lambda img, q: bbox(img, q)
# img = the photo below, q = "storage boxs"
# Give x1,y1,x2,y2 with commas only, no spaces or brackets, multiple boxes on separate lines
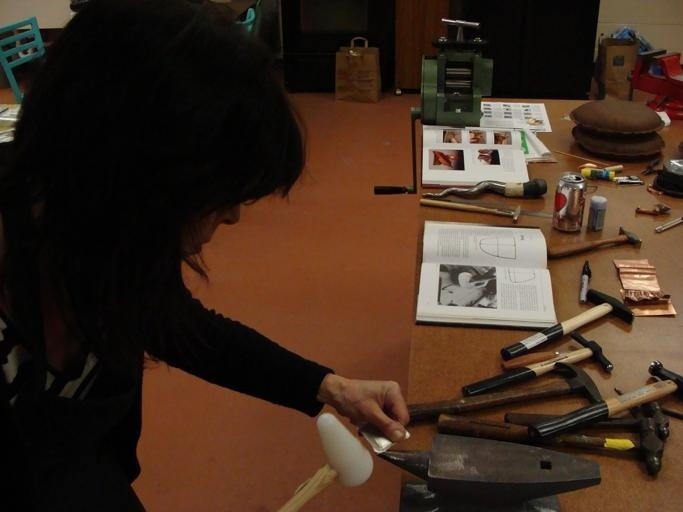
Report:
592,33,683,119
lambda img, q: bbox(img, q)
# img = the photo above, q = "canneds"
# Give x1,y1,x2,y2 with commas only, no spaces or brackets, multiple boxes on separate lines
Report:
552,172,587,232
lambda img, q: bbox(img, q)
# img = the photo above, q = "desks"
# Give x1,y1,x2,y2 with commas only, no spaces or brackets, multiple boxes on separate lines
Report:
404,98,682,510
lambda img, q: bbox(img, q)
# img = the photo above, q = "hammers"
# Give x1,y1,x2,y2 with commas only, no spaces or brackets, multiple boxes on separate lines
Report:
545,226,643,259
500,287,635,362
388,332,683,478
419,197,522,223
277,411,374,512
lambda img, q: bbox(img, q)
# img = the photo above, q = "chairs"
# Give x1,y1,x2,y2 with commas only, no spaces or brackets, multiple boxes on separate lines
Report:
0,16,52,101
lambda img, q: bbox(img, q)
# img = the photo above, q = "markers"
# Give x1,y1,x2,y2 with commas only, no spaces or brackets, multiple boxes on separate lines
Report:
580,259,591,302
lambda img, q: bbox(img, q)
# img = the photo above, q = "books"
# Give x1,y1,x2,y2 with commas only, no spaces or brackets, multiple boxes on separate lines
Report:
416,220,559,330
421,123,530,189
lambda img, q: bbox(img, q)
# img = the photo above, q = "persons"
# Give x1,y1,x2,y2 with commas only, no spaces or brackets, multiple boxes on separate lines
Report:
0,1,415,512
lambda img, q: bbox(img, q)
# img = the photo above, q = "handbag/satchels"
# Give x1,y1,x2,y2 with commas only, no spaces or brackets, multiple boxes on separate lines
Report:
598,32,640,102
334,36,382,104
